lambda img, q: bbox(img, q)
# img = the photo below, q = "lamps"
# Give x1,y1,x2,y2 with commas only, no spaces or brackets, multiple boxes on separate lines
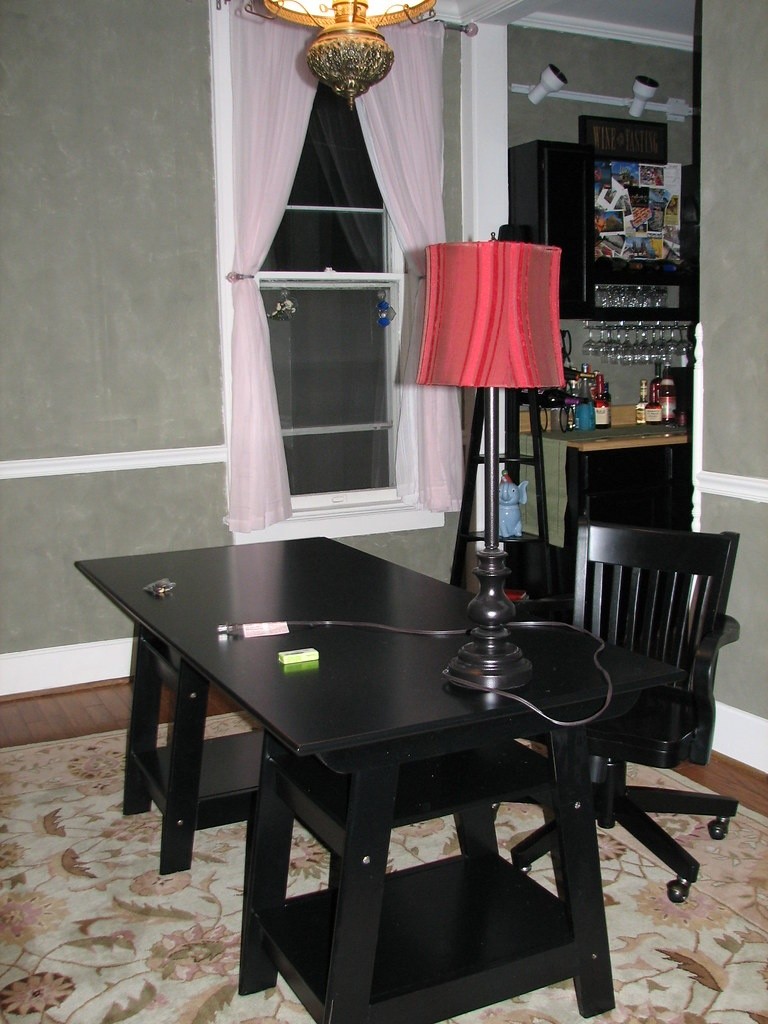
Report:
414,234,565,695
529,64,568,105
629,76,659,116
215,0,439,110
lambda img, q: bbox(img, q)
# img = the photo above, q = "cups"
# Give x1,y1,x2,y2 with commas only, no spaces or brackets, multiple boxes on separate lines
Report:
595,285,668,307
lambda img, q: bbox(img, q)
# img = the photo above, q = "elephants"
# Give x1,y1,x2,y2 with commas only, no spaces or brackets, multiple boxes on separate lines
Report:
498,480,529,538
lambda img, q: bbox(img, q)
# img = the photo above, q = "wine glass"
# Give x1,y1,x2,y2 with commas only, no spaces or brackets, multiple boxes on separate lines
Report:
581,320,693,366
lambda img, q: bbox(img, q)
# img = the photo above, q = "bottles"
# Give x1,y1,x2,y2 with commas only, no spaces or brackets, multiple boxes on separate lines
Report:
650,363,662,404
594,374,609,429
660,364,677,425
636,379,648,424
574,364,596,431
645,382,663,425
603,380,611,427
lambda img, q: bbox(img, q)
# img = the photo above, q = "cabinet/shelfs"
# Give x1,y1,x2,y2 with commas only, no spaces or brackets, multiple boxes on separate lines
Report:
509,137,700,319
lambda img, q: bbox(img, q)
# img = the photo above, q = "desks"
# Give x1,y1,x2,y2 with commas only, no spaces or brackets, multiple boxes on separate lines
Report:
73,534,616,1024
512,428,688,555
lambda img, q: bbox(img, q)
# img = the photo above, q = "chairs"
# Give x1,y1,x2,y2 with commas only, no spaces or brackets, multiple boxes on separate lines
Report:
508,520,740,903
